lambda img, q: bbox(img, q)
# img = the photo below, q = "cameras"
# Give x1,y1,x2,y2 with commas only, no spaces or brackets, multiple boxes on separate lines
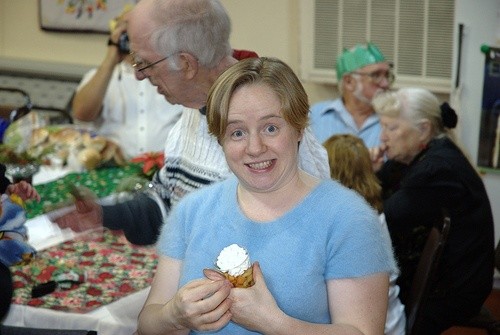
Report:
118,31,130,55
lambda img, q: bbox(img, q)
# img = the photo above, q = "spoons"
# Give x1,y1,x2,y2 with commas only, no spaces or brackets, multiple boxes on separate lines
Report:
203,268,234,288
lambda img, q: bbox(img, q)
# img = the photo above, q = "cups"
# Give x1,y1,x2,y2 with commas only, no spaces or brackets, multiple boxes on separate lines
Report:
214,253,255,288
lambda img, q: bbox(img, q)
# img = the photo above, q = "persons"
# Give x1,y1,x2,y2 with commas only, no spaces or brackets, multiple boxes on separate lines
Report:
320,132,407,334
136,56,398,334
364,85,494,334
72,7,188,163
305,40,396,164
42,1,331,248
0,160,42,205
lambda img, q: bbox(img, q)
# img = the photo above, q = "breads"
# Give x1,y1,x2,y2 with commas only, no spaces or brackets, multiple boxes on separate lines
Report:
12,109,127,170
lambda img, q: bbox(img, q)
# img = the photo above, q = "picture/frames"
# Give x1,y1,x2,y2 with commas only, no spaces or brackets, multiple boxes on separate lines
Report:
37,0,146,34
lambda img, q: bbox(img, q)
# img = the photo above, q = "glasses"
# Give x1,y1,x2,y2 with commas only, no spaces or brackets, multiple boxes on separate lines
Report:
346,69,394,82
128,50,180,73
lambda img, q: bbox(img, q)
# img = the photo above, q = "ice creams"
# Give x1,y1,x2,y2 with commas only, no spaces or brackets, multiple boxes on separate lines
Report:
216,243,253,288
